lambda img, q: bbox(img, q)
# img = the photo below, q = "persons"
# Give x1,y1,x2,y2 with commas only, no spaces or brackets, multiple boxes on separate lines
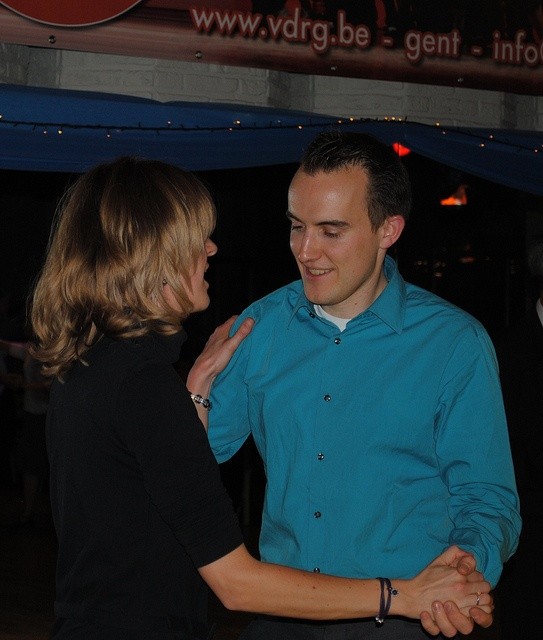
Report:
29,154,494,640
208,131,523,640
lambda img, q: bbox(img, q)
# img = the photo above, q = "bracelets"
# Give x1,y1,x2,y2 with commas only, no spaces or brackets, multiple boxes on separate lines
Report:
185,388,212,410
377,577,393,628
